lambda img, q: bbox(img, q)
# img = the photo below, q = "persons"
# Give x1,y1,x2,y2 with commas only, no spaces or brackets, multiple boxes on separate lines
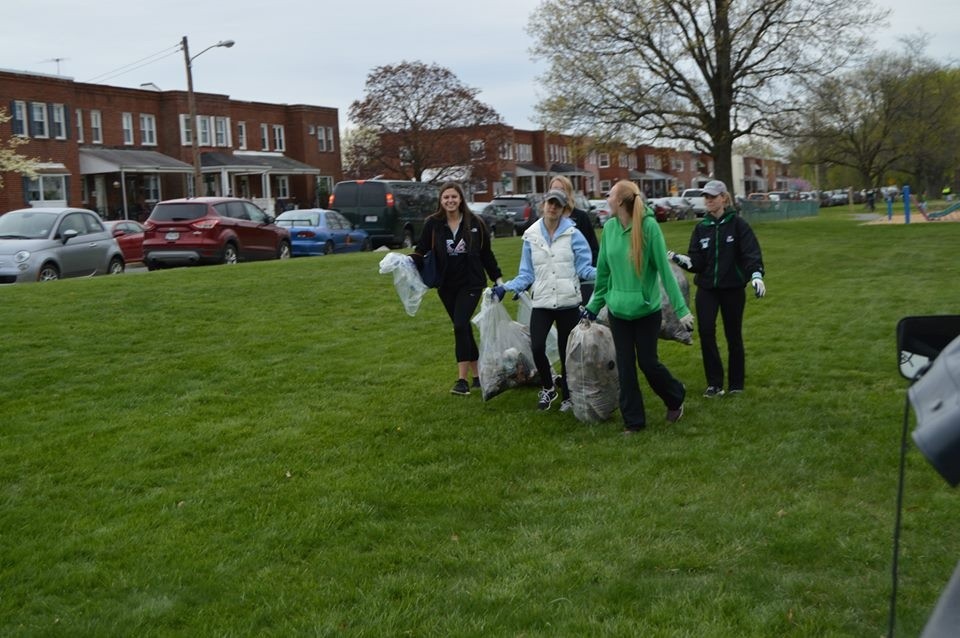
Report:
491,176,765,432
389,183,506,395
866,189,876,212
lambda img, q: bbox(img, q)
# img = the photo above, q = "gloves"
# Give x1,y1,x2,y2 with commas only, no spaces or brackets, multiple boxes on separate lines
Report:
491,286,506,302
752,278,766,298
667,251,683,265
581,309,596,320
678,313,695,331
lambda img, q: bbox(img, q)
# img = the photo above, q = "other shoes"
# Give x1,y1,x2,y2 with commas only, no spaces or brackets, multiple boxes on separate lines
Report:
666,383,685,423
622,426,633,433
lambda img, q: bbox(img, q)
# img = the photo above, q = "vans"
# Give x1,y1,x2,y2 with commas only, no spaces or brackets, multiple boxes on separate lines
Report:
327,179,442,250
681,189,707,215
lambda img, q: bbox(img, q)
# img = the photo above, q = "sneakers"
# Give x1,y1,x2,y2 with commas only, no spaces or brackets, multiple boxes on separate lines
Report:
730,389,742,394
703,386,725,398
472,382,480,388
537,385,559,411
450,379,471,395
560,398,573,412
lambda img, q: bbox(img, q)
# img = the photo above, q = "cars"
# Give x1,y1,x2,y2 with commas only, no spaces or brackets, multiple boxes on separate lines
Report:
491,195,541,236
466,202,517,238
103,219,145,263
274,207,371,256
747,193,772,212
768,191,849,211
647,198,697,222
587,200,613,228
143,196,292,272
0,208,125,284
576,195,598,226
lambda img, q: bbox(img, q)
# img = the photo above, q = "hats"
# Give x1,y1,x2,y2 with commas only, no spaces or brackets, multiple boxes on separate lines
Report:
543,189,569,207
700,180,727,196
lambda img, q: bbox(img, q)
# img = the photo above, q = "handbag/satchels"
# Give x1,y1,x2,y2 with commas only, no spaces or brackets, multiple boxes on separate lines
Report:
421,229,439,289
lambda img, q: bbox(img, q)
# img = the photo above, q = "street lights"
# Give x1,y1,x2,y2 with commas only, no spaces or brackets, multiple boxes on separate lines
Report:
185,40,235,197
